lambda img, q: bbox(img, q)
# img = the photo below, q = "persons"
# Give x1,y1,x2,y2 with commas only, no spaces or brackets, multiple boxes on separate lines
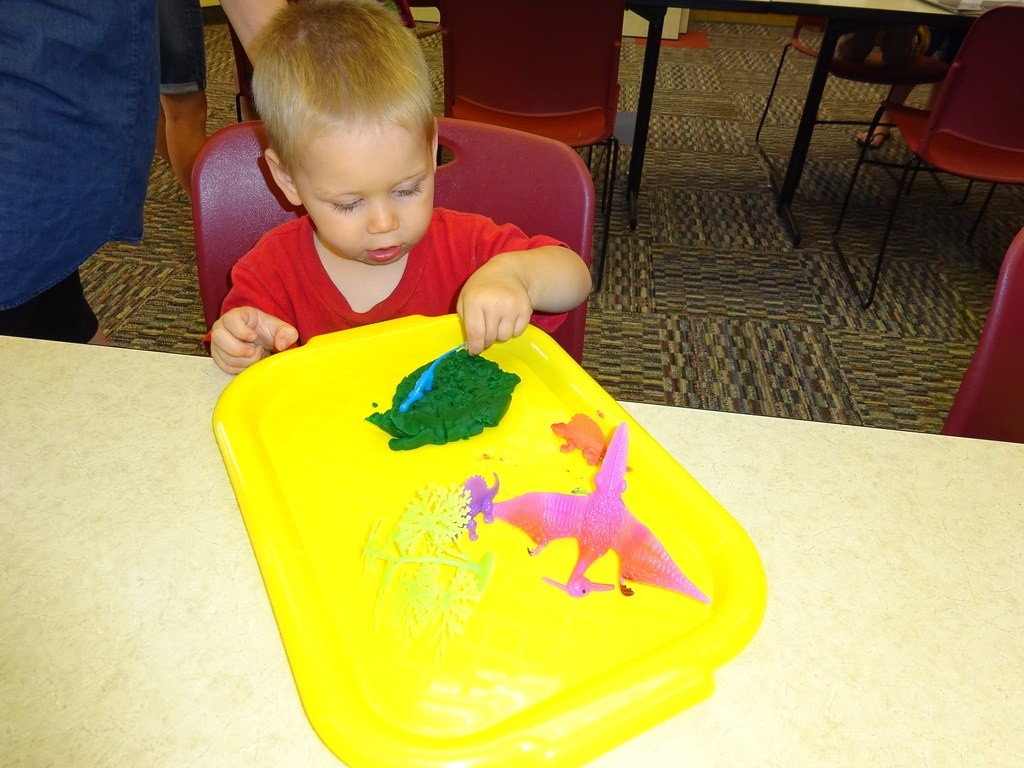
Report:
211,0,593,377
0,0,290,344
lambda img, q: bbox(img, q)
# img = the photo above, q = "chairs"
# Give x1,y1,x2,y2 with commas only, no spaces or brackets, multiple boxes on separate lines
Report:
190,0,1024,443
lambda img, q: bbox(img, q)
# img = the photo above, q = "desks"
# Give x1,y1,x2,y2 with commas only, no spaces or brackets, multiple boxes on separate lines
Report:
0,327,1024,768
618,0,981,243
